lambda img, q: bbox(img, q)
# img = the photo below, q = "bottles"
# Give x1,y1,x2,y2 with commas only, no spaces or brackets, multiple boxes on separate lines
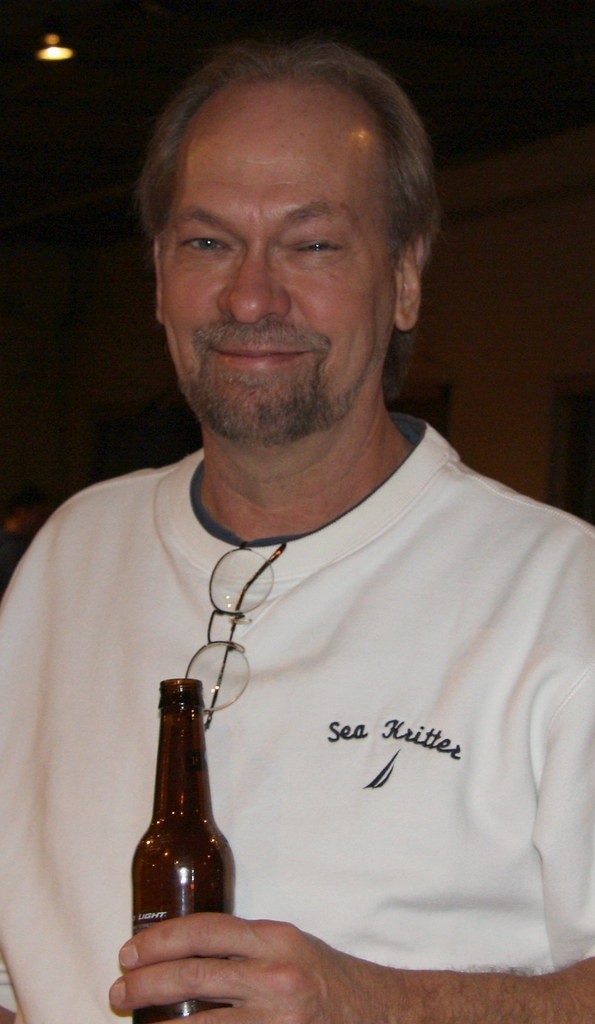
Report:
128,678,237,1024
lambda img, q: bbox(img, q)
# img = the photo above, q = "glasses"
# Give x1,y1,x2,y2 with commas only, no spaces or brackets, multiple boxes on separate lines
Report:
184,538,288,730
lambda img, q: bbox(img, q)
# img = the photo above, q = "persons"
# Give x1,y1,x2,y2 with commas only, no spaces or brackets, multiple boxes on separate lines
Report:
0,38,595,1024
0,490,46,598
